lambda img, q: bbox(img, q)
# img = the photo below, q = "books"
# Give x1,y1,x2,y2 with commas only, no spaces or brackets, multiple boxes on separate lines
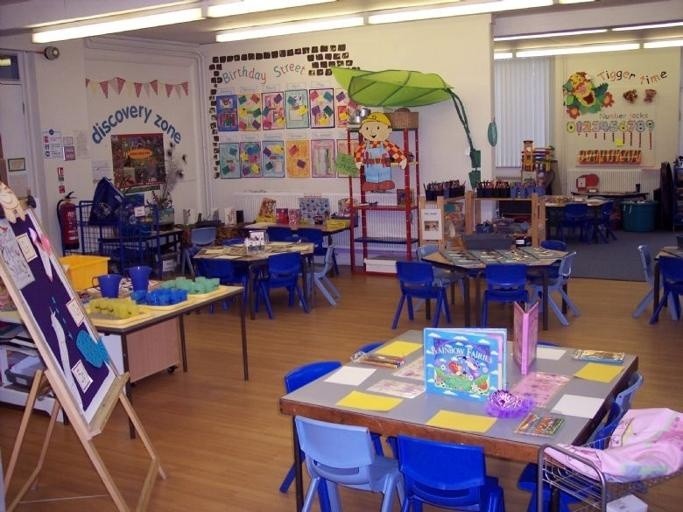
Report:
513,301,539,376
423,326,508,404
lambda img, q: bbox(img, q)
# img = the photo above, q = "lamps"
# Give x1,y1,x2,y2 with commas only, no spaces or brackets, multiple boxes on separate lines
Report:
27,0,366,43
370,0,591,27
493,24,682,61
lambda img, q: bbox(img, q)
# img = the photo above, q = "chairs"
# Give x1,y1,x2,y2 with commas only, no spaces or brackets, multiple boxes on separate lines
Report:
516,370,645,509
185,227,341,321
357,342,383,355
392,236,580,325
546,197,618,245
280,361,506,512
634,245,683,322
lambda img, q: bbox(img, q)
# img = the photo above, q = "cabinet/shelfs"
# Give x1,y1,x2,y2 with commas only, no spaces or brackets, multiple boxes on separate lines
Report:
342,125,419,270
98,226,182,280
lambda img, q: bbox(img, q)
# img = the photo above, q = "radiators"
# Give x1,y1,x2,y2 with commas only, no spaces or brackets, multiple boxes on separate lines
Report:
564,169,644,196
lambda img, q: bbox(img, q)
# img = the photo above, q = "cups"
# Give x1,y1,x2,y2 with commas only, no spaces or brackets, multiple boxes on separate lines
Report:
91,275,121,298
635,183,639,192
129,287,187,305
510,187,544,201
443,188,449,198
160,276,220,294
123,266,151,292
89,298,136,319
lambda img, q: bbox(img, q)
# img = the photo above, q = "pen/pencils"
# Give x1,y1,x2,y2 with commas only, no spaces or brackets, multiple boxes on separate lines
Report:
476,180,507,189
360,357,404,369
423,180,466,191
518,412,564,436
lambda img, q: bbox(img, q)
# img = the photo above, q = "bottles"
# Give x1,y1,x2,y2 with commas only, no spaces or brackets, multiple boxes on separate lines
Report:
516,236,525,250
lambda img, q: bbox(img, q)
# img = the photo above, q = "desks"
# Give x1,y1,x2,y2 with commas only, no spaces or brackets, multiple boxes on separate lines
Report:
0,277,248,439
279,330,639,512
244,217,351,275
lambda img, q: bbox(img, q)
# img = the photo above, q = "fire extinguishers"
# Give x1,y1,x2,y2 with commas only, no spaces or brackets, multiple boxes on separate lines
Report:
56,191,80,250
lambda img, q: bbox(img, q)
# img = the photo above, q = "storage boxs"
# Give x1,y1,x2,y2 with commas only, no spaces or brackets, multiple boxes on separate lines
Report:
57,253,109,292
